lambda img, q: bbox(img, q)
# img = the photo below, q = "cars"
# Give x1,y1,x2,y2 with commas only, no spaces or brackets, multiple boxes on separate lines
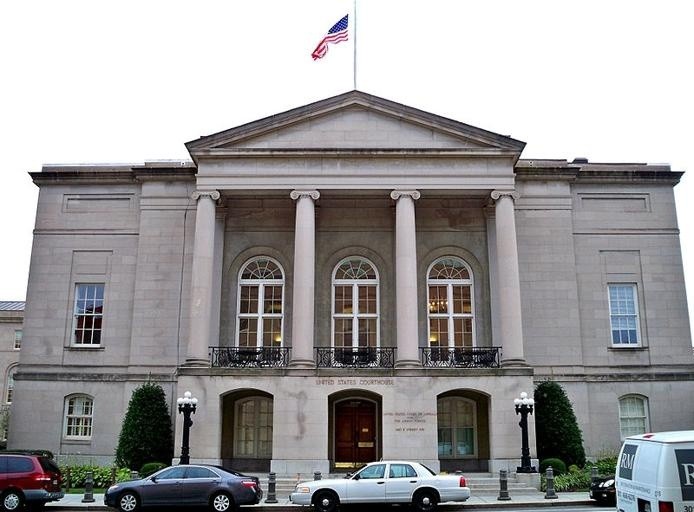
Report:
103,464,263,512
590,476,616,506
290,460,470,510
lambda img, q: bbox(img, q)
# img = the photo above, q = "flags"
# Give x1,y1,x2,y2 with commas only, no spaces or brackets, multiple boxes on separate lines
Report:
311,13,349,61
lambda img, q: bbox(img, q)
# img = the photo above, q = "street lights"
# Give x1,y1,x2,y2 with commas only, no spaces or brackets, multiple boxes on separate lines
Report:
176,392,198,464
513,392,534,466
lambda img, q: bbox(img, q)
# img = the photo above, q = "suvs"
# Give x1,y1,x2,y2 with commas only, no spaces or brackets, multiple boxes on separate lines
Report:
0,452,65,511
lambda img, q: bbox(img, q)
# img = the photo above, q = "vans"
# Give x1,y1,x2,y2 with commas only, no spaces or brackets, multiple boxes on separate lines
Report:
614,430,694,511
